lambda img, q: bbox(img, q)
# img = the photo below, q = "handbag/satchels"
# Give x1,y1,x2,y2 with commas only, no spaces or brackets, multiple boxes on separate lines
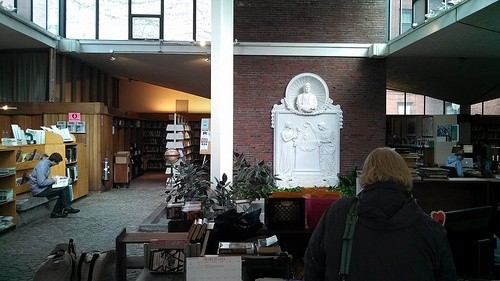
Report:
212,207,264,242
73,250,116,281
33,239,78,281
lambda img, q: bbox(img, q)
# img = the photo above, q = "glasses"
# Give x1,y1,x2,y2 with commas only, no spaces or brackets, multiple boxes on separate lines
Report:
54,161,59,164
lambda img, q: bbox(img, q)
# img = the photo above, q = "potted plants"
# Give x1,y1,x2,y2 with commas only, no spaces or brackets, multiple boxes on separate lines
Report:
166,151,283,224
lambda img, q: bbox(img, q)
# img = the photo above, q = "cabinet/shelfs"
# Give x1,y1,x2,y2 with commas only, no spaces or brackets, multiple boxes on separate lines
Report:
143,121,206,169
126,120,143,176
115,228,190,281
387,143,433,167
114,164,132,188
164,114,192,192
0,130,90,236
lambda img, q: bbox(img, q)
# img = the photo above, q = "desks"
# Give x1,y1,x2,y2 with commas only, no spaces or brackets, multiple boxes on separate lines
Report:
394,166,500,281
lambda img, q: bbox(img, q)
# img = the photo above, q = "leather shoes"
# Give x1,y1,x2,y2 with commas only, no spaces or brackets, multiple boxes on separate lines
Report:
64,207,80,214
51,212,67,217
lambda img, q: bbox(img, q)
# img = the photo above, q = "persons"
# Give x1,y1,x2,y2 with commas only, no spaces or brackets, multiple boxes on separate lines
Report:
475,155,492,170
307,121,335,179
296,82,317,113
279,121,298,180
30,153,80,219
304,147,448,281
445,153,464,176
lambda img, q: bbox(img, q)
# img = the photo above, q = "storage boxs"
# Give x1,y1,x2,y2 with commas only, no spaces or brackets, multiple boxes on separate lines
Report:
307,198,339,230
264,193,307,229
116,151,131,164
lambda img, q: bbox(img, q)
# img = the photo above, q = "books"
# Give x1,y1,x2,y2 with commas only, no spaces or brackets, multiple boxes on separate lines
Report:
0,187,14,202
165,200,202,220
142,121,166,167
178,121,209,161
187,218,208,240
0,167,16,177
393,148,450,180
69,185,73,201
65,147,78,181
40,124,74,142
1,125,46,146
219,235,280,256
52,176,69,187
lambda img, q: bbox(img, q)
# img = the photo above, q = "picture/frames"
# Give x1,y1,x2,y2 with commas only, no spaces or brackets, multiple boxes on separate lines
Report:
393,117,460,142
